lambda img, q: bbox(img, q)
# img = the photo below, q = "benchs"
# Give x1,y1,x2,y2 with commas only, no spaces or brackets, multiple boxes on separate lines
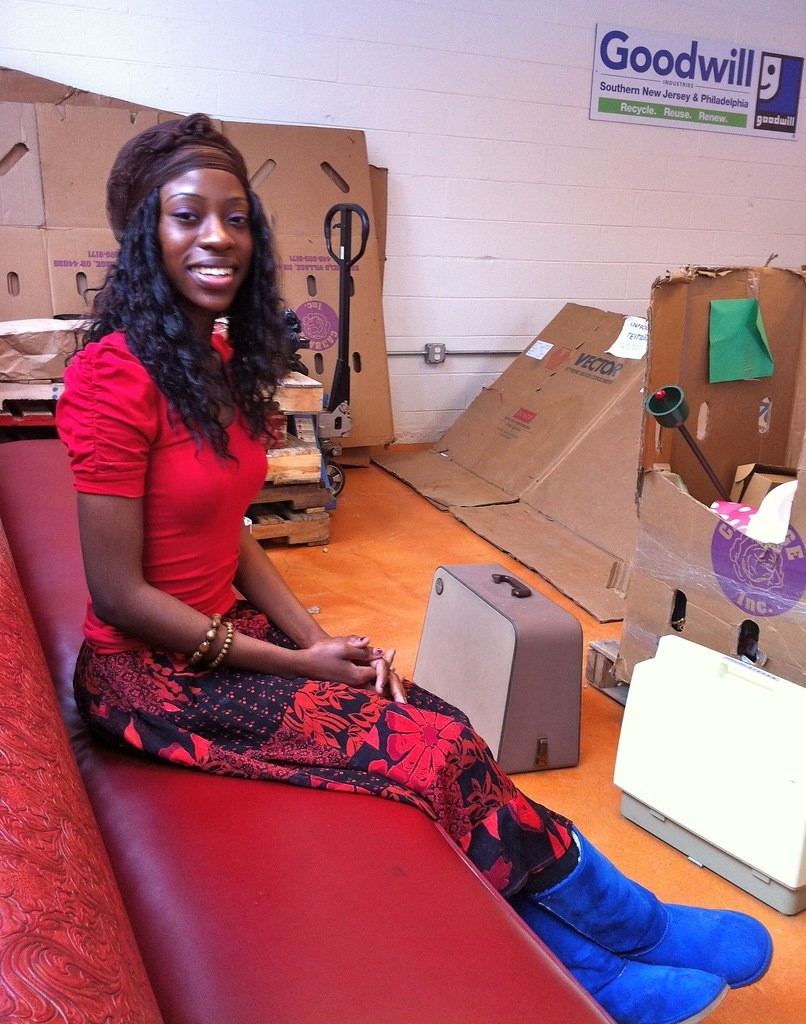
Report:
0,435,622,1024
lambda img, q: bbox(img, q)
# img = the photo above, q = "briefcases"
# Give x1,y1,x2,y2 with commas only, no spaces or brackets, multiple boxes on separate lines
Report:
413,564,584,773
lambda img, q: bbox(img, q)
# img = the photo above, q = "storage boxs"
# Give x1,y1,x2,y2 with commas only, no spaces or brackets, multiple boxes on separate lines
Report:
612,462,806,687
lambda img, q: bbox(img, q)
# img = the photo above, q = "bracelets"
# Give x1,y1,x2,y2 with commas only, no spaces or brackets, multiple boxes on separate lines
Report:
190,611,234,669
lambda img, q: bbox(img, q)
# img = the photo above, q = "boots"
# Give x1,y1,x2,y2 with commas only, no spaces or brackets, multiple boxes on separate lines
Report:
516,902,729,1024
532,825,774,988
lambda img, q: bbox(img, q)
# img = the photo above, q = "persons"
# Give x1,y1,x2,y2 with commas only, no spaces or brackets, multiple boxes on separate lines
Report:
55,113,775,1024
